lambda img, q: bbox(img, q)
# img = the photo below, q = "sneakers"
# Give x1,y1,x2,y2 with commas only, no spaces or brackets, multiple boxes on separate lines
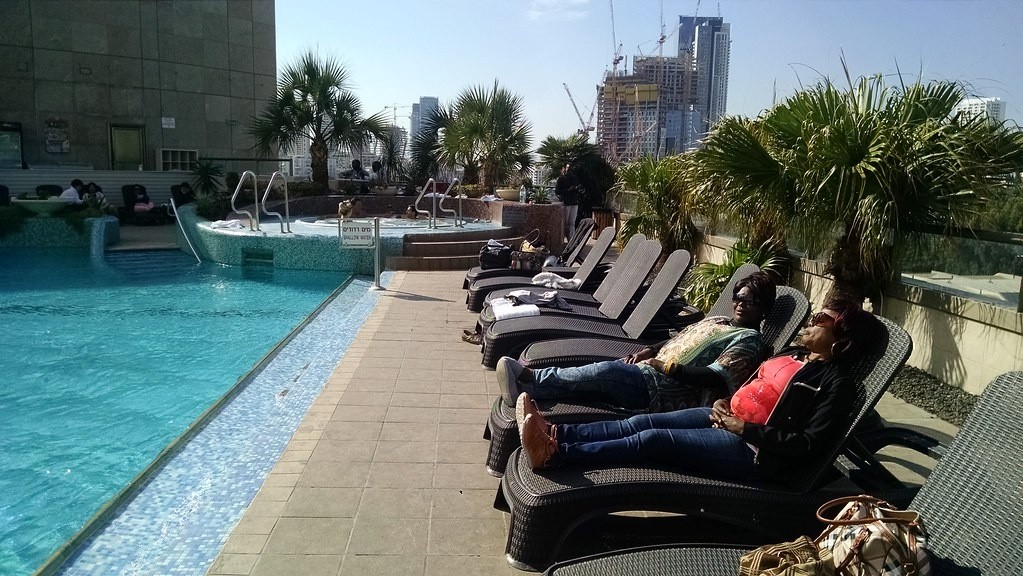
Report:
519,413,555,469
515,391,549,448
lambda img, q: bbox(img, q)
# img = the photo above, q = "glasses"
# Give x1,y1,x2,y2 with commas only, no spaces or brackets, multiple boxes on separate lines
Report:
811,312,835,326
730,294,761,306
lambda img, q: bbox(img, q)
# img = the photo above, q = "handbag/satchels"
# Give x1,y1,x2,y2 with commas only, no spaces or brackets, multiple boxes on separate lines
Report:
520,229,551,254
479,243,513,269
510,250,542,271
814,492,931,576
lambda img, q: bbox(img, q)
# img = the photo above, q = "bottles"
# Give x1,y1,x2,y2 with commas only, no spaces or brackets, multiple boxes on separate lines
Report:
519,184,526,203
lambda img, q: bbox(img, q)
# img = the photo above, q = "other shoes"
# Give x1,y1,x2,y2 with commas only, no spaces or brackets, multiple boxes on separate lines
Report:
461,324,483,344
500,356,527,385
496,358,517,407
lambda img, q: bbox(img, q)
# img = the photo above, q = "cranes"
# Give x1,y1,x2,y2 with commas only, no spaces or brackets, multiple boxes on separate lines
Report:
558,0,724,142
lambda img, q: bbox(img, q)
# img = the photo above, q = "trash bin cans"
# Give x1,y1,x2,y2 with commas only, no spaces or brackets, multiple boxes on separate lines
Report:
591,206,614,241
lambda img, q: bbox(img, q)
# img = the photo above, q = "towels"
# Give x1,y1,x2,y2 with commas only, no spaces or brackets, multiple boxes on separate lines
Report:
489,295,542,321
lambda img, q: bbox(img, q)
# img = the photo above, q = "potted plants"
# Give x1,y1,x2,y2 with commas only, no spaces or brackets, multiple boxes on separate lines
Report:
243,174,258,203
465,183,485,198
496,177,520,200
372,182,397,196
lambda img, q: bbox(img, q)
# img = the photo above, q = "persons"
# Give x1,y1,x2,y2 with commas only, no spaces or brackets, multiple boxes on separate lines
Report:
515,296,873,485
337,197,419,223
494,271,779,413
124,185,155,212
309,163,315,183
337,159,370,180
365,161,386,181
81,181,118,217
554,163,585,242
168,182,193,215
58,179,85,206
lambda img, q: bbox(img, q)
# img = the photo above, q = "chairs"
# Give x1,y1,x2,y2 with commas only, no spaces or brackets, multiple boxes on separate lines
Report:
463,217,599,290
465,225,617,313
492,312,915,574
36,184,63,196
475,240,664,342
516,263,761,370
482,233,647,307
483,285,812,478
171,184,194,197
480,248,691,369
121,184,166,226
542,366,1023,576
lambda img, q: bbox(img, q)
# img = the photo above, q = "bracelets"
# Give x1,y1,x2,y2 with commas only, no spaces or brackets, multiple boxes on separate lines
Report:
660,362,674,376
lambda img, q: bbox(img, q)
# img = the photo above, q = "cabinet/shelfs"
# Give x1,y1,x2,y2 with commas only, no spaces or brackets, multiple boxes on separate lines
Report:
154,147,199,172
